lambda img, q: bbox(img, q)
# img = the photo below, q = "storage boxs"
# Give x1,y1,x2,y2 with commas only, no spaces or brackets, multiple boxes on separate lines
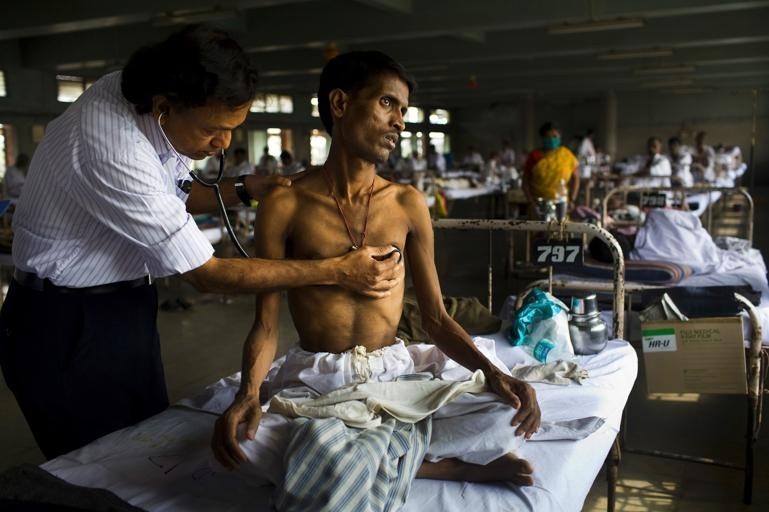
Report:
641,315,749,397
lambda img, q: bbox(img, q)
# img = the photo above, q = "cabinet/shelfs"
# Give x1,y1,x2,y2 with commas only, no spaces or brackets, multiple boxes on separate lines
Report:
506,188,529,217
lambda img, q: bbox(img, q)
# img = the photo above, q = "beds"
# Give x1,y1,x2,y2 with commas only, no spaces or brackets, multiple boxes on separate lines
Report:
514,186,763,506
586,163,705,210
416,169,505,217
0,219,626,512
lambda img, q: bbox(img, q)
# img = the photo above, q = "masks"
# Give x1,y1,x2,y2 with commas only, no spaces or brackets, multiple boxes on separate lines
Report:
544,136,561,149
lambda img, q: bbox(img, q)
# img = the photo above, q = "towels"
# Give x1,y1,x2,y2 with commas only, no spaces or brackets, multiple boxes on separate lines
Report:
511,360,589,386
267,369,485,429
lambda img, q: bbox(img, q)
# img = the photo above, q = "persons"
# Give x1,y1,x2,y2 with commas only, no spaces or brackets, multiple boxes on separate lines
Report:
258,145,277,175
668,137,693,187
693,131,716,182
319,166,377,254
589,207,721,274
490,138,515,175
225,147,255,177
278,149,306,175
412,150,427,172
521,120,580,221
618,135,672,187
426,144,444,172
4,152,32,210
214,49,541,492
206,153,228,179
715,143,731,178
464,146,485,174
576,127,599,180
1,19,405,459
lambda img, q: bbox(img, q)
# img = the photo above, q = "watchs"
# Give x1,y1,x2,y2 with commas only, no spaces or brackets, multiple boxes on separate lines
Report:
235,174,256,209
